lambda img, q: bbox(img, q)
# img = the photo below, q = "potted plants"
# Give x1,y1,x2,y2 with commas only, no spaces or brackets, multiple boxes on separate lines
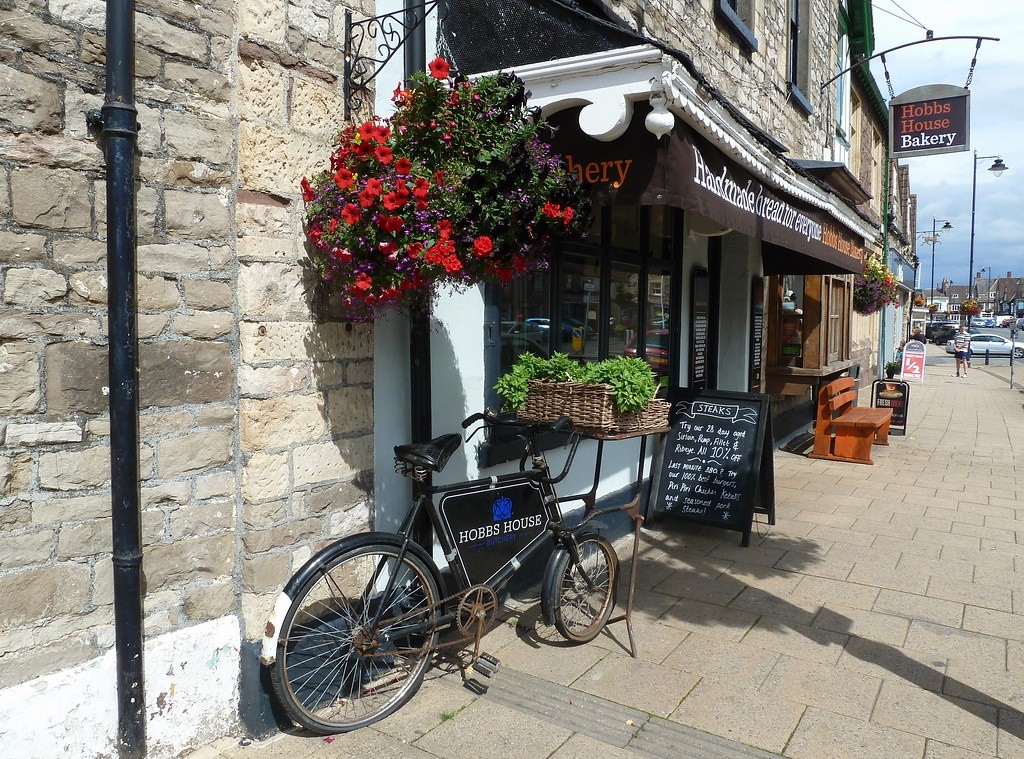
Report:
493,350,671,435
884,360,898,378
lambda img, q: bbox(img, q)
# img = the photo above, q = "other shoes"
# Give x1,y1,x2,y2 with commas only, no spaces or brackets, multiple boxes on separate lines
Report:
951,373,960,377
961,372,967,378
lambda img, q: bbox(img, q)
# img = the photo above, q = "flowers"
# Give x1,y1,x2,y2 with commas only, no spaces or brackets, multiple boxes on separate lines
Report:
300,56,596,331
852,250,899,316
928,304,938,311
915,293,926,306
959,299,984,316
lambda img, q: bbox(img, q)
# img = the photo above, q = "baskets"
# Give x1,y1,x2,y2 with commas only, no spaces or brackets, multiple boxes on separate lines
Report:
515,377,672,434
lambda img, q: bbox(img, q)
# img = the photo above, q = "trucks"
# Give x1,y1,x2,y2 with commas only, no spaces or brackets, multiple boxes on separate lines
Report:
996,315,1014,329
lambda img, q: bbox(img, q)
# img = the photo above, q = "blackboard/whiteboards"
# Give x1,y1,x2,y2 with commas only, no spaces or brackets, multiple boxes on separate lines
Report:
648,385,777,531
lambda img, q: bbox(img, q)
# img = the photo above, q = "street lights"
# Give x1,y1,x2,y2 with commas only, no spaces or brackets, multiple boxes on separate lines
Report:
968,149,1010,299
925,216,955,338
981,267,991,308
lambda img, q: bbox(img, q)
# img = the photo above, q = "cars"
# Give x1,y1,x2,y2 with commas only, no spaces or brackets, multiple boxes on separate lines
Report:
501,312,595,361
970,317,996,329
1001,318,1024,329
933,323,1024,359
623,329,669,375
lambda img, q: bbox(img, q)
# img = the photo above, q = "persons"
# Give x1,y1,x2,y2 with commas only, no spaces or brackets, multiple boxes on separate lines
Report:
909,327,927,345
951,325,971,377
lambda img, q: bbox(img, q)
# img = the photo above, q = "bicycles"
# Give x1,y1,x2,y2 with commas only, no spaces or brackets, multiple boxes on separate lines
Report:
259,406,624,734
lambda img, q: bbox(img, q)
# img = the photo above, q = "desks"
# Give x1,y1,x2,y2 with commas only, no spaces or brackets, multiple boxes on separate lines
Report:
518,426,671,656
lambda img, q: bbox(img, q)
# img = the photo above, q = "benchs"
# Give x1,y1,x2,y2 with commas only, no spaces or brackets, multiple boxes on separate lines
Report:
807,377,894,466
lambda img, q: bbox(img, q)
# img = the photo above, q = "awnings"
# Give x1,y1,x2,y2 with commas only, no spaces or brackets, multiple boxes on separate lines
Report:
435,1,884,277
